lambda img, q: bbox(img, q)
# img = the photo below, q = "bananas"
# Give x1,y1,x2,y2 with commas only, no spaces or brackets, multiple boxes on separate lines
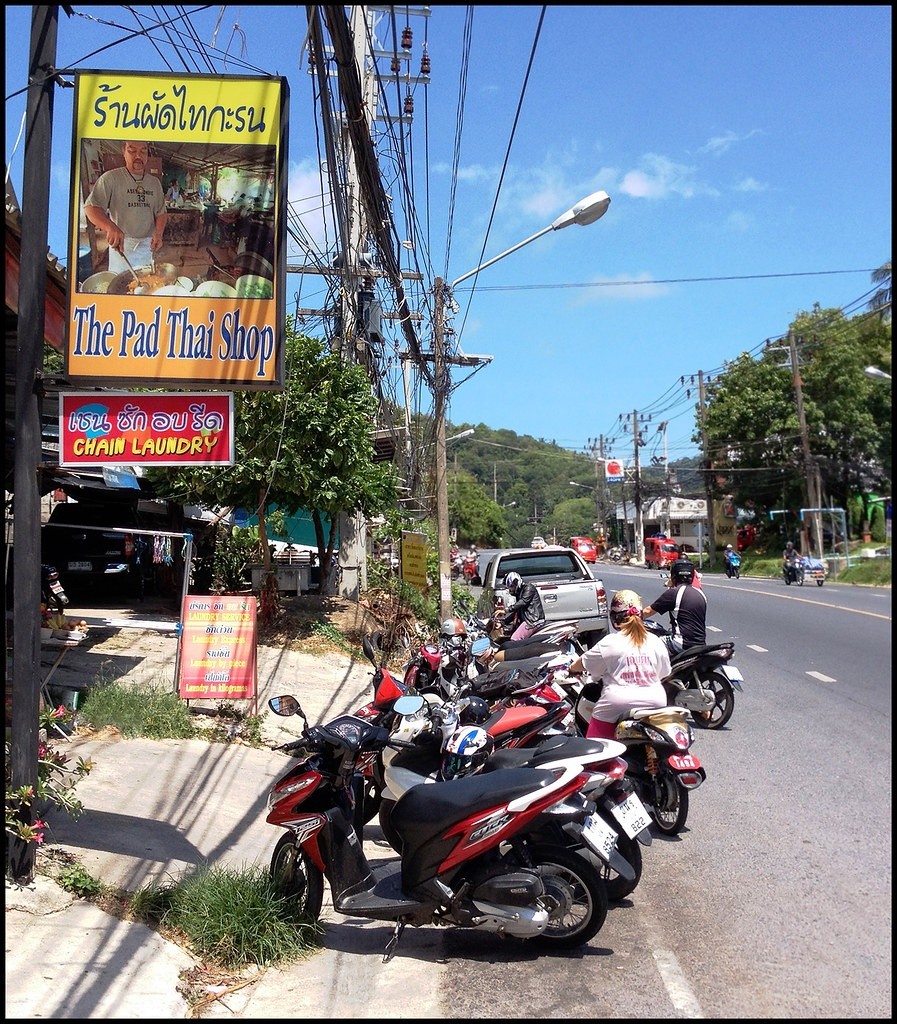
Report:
46,613,67,630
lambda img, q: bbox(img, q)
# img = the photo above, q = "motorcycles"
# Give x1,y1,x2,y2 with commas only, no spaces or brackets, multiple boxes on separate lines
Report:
450,555,480,585
264,596,706,965
642,618,744,730
783,558,825,586
41,564,69,615
726,555,740,579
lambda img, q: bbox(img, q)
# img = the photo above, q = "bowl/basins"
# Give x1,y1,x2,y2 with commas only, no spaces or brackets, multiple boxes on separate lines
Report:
80,270,118,293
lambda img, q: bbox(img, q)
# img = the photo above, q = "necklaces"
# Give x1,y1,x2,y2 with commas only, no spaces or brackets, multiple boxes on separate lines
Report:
126,167,145,193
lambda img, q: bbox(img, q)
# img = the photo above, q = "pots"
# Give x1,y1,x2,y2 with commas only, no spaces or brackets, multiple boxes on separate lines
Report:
107,248,178,295
206,248,260,286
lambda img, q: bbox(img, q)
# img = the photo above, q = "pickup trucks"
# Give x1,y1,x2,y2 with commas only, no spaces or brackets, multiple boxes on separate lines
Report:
470,547,610,638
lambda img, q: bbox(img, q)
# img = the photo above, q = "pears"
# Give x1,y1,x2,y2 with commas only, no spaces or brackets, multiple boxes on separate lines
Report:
62,619,89,632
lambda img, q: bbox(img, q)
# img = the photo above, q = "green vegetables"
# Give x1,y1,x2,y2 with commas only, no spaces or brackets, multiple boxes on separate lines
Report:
241,281,273,299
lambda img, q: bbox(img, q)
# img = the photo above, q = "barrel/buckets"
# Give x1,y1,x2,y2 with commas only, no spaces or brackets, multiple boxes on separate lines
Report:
62,689,80,712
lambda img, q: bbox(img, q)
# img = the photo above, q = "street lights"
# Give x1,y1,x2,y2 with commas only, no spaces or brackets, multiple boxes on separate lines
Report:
402,190,612,623
597,457,644,562
569,481,601,538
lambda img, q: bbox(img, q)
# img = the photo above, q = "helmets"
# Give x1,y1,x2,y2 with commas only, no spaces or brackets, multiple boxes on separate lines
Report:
611,590,643,612
786,542,793,550
727,544,732,549
476,647,494,666
678,554,688,559
441,726,495,781
506,572,523,595
456,696,492,726
471,545,476,550
439,618,467,637
670,559,694,585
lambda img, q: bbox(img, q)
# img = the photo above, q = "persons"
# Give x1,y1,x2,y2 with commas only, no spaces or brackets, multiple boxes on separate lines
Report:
783,541,802,581
217,190,263,209
166,179,187,200
83,141,168,274
463,545,479,579
643,559,714,719
724,544,741,570
493,571,545,641
665,553,702,589
568,589,673,739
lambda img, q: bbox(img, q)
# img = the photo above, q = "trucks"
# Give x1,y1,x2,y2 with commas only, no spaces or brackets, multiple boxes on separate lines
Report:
570,537,597,564
644,537,682,570
